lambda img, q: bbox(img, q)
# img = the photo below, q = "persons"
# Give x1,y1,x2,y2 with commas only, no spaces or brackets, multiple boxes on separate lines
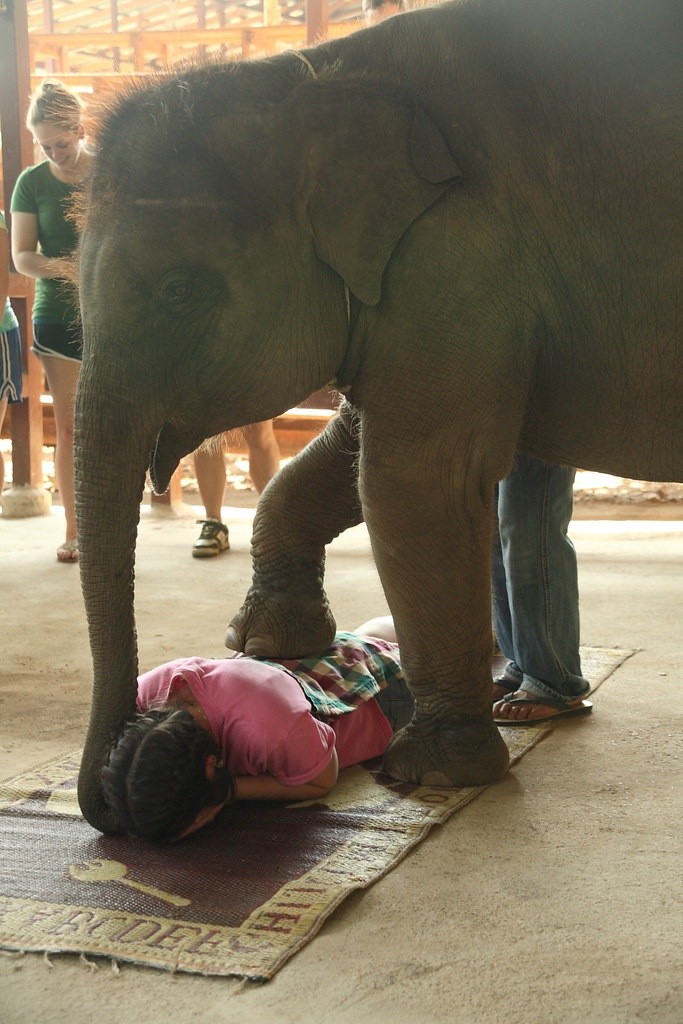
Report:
0,204,25,495
361,0,409,27
13,81,103,563
92,611,417,841
490,447,592,724
191,417,282,558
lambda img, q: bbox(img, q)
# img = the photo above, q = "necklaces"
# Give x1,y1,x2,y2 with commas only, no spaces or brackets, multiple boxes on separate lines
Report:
184,699,209,720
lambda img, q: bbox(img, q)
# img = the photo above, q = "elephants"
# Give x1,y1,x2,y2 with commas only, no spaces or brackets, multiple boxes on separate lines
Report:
38,2,683,842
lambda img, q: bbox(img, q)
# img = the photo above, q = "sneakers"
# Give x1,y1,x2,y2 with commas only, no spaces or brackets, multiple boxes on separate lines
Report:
192,519,231,557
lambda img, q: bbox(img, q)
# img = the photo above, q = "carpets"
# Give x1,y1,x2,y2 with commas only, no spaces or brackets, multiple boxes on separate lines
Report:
0,622,643,982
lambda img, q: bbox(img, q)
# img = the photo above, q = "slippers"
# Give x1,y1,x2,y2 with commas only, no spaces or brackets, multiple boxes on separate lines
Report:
57,539,79,562
491,675,594,727
